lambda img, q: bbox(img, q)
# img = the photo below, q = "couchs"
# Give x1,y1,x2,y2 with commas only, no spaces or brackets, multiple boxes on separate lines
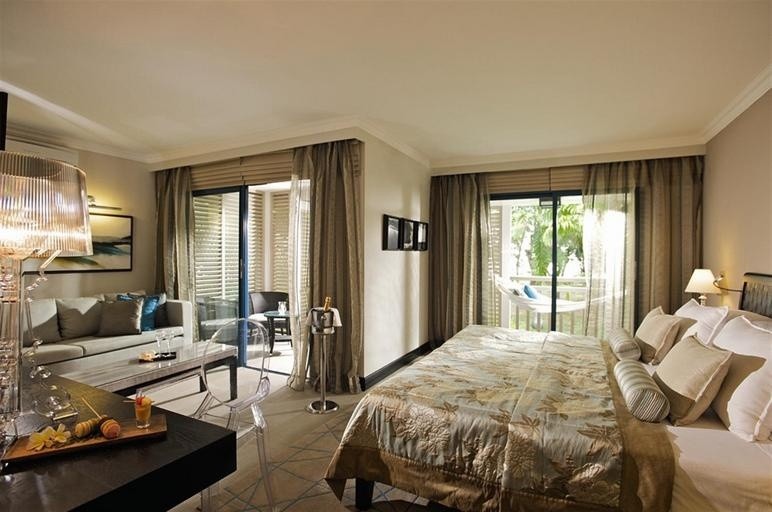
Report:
23,289,194,374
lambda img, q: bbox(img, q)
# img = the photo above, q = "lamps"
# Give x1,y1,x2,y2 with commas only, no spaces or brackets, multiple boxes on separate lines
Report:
685,269,723,307
711,273,747,295
0,150,93,446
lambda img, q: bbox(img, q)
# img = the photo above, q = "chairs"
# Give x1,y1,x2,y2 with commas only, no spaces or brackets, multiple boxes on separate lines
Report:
187,316,275,512
194,295,241,344
247,290,289,345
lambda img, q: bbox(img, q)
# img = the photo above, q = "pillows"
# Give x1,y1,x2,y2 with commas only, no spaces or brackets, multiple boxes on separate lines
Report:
117,294,159,332
96,299,144,338
510,281,539,309
607,296,772,452
128,292,166,328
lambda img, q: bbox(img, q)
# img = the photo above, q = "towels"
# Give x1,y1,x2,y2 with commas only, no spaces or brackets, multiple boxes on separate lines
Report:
304,305,343,328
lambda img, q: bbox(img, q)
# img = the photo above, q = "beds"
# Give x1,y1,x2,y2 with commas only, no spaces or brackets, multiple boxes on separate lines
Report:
327,270,772,512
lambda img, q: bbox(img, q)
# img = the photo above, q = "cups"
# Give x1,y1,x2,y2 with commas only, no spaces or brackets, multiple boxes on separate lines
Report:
134,403,151,427
278,301,287,315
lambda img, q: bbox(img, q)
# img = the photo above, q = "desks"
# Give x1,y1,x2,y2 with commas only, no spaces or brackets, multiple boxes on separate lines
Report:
0,370,237,512
263,310,292,355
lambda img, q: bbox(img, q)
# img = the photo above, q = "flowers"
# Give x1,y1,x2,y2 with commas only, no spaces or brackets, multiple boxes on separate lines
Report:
24,424,72,454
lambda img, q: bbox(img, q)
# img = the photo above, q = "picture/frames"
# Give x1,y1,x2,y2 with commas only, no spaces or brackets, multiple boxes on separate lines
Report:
383,214,427,251
22,212,133,273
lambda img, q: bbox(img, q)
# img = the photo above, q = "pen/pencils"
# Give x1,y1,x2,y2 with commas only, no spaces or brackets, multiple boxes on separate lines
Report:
122,400,155,403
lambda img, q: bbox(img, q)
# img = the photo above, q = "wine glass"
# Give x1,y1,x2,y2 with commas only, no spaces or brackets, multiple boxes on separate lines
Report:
153,329,166,357
163,329,175,357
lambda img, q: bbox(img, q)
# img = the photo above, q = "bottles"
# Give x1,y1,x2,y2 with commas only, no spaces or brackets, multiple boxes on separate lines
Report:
323,296,332,312
